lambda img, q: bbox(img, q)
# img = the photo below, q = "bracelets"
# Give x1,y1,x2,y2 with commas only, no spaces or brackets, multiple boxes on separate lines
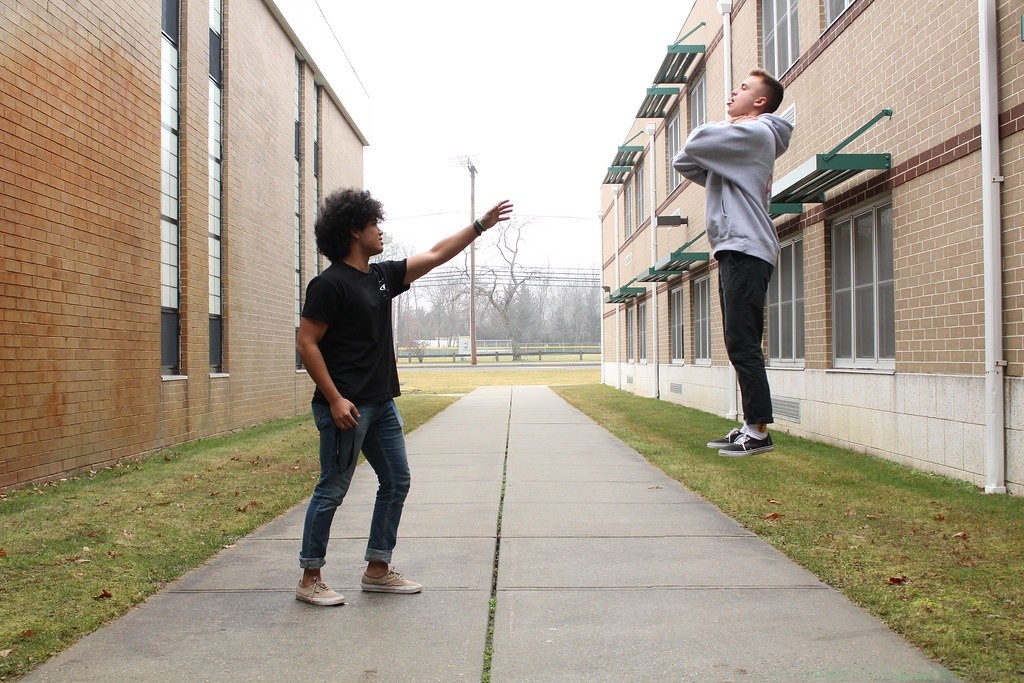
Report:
473,219,486,236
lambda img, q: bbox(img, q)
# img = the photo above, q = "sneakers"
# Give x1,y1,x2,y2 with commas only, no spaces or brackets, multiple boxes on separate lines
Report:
707,427,774,457
295,577,346,605
361,569,423,593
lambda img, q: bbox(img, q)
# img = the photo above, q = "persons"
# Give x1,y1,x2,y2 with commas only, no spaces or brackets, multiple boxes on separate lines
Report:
672,68,795,461
294,186,514,606
